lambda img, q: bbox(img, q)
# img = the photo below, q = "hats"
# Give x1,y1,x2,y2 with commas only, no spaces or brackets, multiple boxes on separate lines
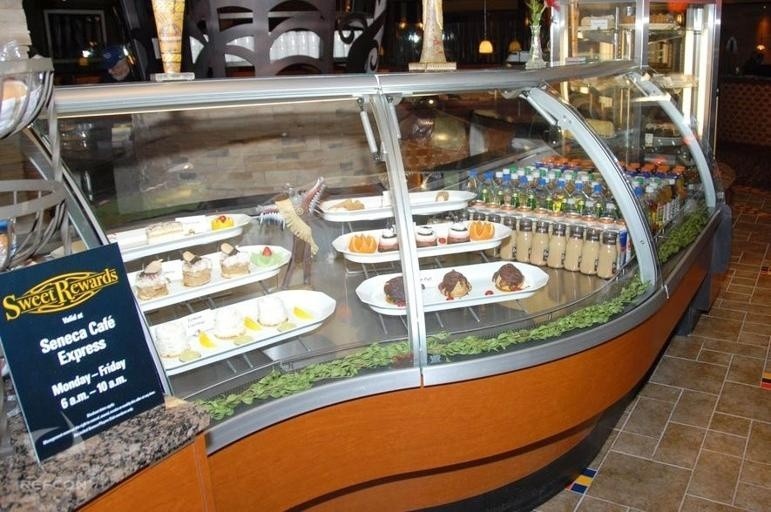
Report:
99,43,129,70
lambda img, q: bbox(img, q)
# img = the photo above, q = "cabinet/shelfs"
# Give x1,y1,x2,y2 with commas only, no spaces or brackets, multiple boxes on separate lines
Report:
551,0,721,179
0,61,723,512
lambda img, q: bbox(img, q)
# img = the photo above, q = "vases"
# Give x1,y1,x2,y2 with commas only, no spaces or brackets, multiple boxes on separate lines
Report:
525,25,547,70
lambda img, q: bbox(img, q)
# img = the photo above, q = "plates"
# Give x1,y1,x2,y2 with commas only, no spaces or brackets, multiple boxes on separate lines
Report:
317,190,478,223
128,243,292,311
147,288,337,375
105,213,250,262
330,221,512,265
355,260,550,317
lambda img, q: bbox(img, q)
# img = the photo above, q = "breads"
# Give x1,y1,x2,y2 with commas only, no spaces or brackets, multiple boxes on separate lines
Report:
0,80,27,103
0,234,10,246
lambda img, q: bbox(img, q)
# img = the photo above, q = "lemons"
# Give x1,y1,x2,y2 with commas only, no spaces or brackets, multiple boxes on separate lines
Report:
293,307,309,319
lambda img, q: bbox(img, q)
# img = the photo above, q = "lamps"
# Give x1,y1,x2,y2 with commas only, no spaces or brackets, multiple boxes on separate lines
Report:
479,0,493,54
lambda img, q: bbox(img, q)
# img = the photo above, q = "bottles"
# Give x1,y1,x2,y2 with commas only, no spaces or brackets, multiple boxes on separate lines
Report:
466,157,686,278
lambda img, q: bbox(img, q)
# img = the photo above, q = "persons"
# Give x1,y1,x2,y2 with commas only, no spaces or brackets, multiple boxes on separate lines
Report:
98,46,139,83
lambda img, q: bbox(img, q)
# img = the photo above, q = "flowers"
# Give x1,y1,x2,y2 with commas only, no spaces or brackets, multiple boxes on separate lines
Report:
524,0,560,58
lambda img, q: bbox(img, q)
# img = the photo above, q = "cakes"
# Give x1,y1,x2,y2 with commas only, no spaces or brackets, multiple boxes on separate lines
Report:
134,216,288,358
329,190,525,308
